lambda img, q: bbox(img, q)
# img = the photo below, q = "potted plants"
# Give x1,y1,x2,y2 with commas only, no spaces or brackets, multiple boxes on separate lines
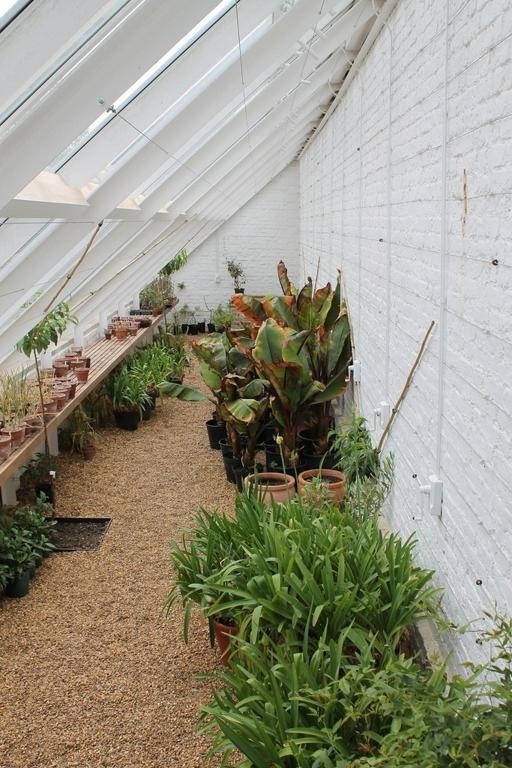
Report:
93,332,190,432
172,304,192,335
1,364,58,464
1,452,61,598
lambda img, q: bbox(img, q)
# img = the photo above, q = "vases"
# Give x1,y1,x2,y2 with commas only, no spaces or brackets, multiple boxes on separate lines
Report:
105,316,152,339
53,346,91,412
